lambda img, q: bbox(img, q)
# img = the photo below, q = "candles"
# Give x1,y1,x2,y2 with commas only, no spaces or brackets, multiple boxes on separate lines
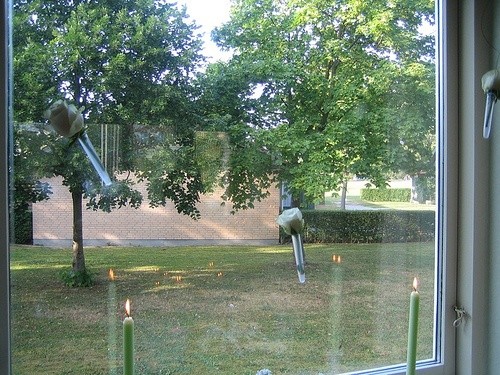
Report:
122,299,135,374
406,276,420,375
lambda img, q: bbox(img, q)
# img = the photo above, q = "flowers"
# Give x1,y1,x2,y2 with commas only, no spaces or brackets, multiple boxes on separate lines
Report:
48,100,106,173
481,69,500,128
275,206,306,274
